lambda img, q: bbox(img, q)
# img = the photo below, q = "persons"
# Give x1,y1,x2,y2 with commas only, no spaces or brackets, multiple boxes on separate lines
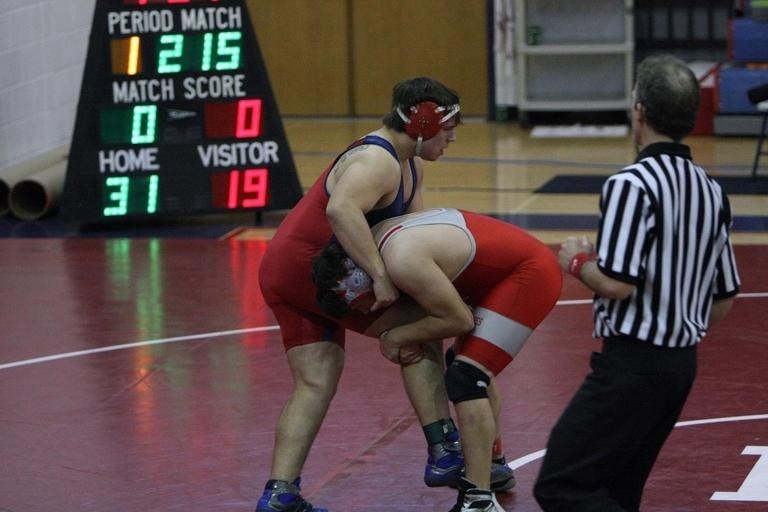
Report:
529,55,745,511
311,206,562,511
256,76,516,512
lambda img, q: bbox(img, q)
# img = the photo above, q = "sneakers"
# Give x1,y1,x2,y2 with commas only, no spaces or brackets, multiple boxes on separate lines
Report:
490,456,517,492
424,432,517,492
447,476,506,512
255,476,329,512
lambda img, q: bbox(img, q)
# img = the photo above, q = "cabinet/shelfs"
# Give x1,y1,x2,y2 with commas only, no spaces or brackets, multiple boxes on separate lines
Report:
510,0,638,133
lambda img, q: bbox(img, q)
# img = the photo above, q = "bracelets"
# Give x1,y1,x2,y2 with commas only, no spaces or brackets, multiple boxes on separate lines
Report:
568,251,597,280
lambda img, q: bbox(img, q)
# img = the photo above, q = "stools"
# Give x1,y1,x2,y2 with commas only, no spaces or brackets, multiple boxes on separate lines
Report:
746,82,766,178
